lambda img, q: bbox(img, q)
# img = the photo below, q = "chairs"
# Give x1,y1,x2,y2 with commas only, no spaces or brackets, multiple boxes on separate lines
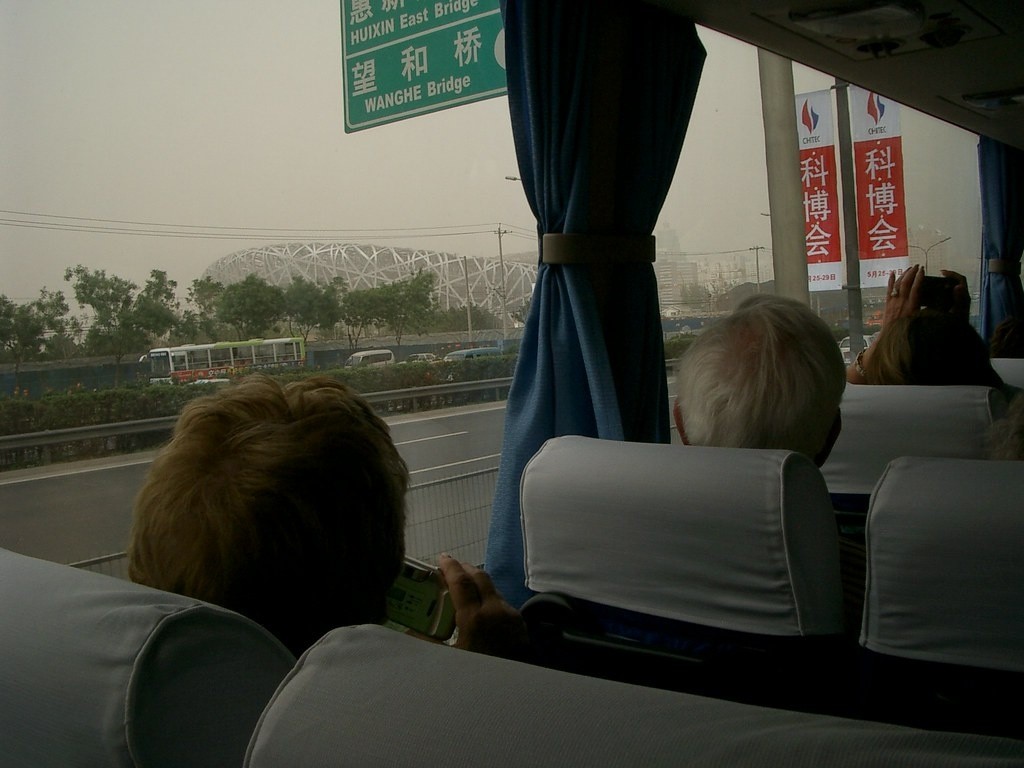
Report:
0,358,1024,768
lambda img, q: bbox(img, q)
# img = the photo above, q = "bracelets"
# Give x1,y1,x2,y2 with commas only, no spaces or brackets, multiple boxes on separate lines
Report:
855,348,872,378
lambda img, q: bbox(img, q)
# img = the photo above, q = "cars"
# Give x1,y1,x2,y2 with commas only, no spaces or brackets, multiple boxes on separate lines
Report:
396,352,438,364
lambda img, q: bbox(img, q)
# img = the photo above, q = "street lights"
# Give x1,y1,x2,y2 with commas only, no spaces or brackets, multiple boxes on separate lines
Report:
909,236,953,276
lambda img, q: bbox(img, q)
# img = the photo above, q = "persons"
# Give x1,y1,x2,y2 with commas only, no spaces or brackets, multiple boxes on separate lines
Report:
672,294,869,638
125,370,536,666
846,264,1024,459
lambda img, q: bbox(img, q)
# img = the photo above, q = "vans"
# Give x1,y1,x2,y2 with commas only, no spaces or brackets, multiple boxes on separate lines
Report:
840,335,876,364
445,347,504,362
344,349,396,369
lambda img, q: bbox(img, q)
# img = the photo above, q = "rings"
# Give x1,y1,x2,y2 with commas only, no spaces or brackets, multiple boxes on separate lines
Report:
890,287,898,296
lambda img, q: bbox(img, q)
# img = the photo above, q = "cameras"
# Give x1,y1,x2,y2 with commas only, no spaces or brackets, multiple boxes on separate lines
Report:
386,554,455,642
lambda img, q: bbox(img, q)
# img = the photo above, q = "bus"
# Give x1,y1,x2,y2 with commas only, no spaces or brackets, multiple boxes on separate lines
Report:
138,336,307,387
